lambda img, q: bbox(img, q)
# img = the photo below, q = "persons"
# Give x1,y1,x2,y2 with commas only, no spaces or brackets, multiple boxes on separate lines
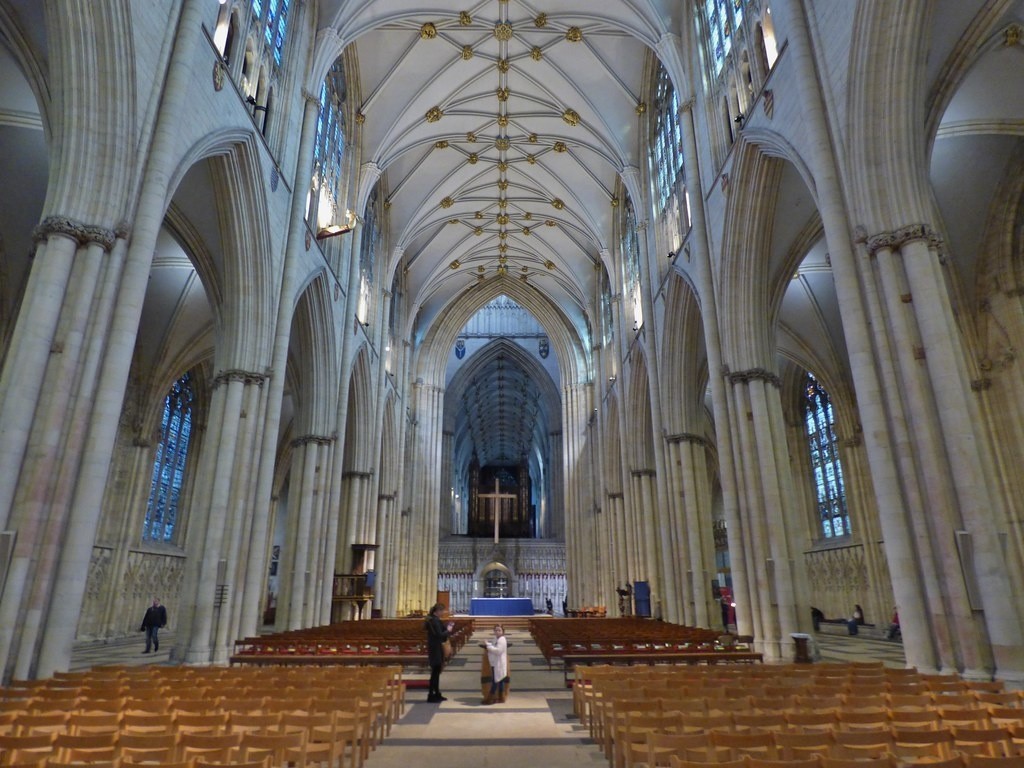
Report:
562,601,567,615
887,607,900,639
721,597,729,633
140,597,167,653
481,624,508,704
810,607,825,632
848,604,864,636
424,603,452,702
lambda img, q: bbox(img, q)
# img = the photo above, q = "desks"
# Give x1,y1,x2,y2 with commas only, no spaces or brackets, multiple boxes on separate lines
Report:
470,598,536,615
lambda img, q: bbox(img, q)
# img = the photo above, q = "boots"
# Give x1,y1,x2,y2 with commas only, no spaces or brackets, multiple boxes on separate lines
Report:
495,691,505,703
480,693,494,704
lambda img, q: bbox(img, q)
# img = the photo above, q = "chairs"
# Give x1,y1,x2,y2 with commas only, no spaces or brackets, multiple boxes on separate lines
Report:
528,616,1023,768
1,618,476,768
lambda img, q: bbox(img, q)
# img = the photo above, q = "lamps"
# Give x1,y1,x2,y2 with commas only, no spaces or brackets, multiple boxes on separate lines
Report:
245,96,257,105
633,327,638,331
735,114,744,122
667,252,675,257
362,323,370,327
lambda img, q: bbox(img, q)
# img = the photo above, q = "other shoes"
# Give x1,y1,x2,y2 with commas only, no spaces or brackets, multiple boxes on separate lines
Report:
428,694,440,702
436,693,447,701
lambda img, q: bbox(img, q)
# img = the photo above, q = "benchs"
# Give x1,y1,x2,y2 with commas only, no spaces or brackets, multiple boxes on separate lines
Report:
817,621,875,627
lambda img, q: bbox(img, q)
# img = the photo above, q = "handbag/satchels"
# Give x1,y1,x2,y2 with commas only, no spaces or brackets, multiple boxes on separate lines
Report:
441,638,451,658
